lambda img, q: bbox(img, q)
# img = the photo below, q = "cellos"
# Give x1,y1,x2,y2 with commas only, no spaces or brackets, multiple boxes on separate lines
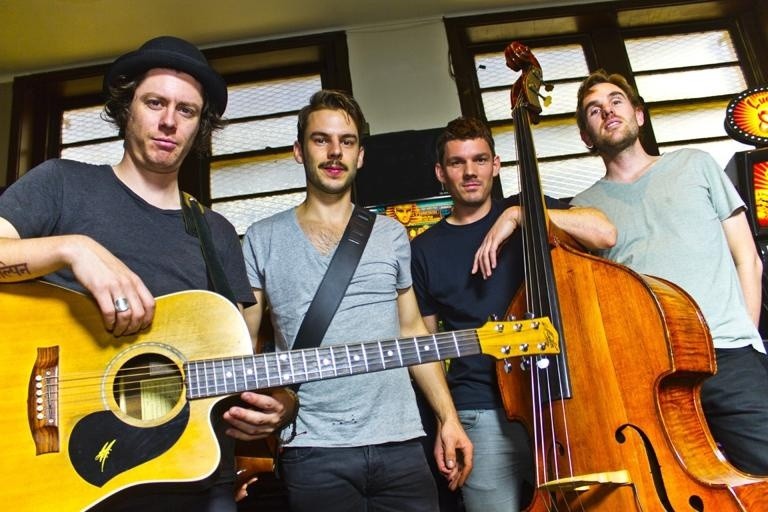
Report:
494,43,766,512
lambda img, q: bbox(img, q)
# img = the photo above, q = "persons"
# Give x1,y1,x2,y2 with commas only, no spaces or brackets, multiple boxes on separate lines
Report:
409,111,622,512
0,31,304,512
563,66,768,476
233,87,478,512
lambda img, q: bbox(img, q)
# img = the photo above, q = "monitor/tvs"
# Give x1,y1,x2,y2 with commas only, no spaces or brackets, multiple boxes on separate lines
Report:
351,127,452,210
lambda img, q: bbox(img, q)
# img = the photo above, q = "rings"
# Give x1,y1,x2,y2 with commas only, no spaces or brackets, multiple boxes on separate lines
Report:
112,296,132,314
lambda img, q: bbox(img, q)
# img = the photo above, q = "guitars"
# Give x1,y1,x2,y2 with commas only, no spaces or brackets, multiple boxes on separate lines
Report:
0,278,559,511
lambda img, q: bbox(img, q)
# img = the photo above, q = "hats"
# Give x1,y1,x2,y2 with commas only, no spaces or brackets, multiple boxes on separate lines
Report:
102,36,229,133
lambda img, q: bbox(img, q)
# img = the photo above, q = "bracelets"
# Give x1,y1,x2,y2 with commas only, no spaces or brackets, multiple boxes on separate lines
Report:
282,387,299,424
